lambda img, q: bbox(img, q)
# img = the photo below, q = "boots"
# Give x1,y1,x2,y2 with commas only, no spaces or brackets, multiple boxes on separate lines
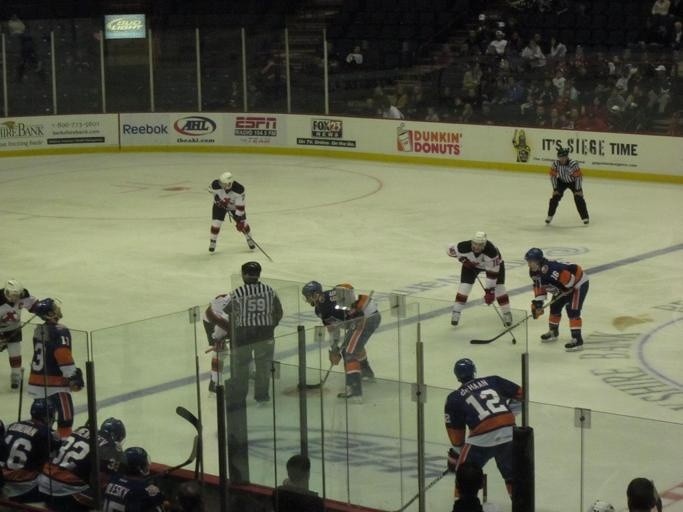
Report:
338,382,362,397
541,323,559,339
565,329,583,348
210,380,217,393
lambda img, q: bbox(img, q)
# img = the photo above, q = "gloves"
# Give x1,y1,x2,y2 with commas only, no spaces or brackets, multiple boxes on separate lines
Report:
485,288,495,304
531,299,544,318
458,256,474,269
236,220,244,231
448,448,460,473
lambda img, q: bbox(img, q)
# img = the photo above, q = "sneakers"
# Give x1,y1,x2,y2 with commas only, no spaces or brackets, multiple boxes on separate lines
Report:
503,311,511,327
11,373,20,388
247,239,255,249
583,218,589,224
545,215,552,223
451,310,460,325
209,239,216,251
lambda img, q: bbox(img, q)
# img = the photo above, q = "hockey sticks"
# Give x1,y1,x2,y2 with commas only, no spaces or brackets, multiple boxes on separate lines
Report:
226,210,274,263
467,263,516,345
176,406,203,474
298,291,373,388
470,290,573,343
161,436,198,472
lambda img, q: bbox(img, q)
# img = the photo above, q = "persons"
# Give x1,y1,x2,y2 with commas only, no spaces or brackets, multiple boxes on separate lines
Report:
451,497,483,512
447,232,513,327
302,282,381,397
223,261,282,401
271,454,322,512
546,150,589,225
202,294,231,391
207,172,255,251
524,247,589,349
627,479,656,512
512,130,531,163
444,358,522,499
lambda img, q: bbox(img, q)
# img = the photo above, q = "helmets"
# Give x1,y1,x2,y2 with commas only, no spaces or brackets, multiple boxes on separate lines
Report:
219,172,234,183
34,298,54,316
242,262,261,275
120,447,150,475
4,279,23,302
454,359,475,379
30,398,55,417
558,149,567,156
302,280,322,295
472,231,487,245
101,418,126,442
525,247,543,259
